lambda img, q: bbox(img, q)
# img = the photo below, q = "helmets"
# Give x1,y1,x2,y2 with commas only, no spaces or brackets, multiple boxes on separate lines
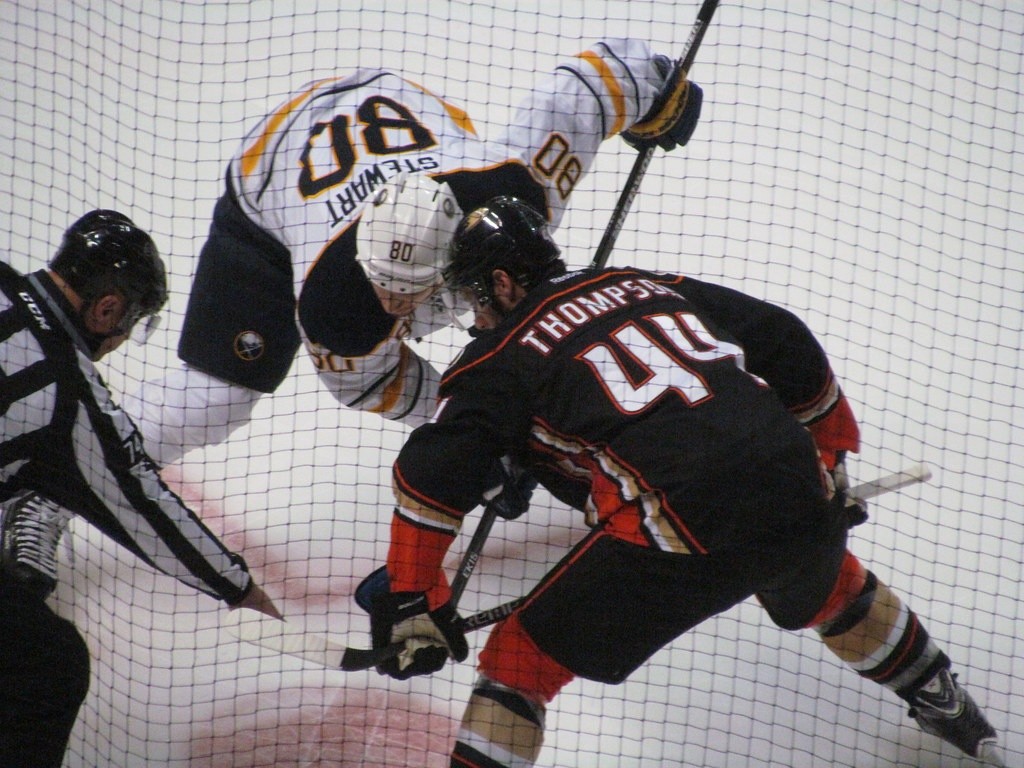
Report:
442,196,559,301
356,171,464,297
50,209,169,341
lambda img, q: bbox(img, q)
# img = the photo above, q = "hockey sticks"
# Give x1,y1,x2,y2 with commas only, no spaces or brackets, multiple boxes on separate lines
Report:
446,0,723,617
220,460,934,673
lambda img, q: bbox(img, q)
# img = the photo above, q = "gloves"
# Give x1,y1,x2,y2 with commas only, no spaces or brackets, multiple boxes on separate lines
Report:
355,562,468,682
827,450,870,527
618,53,704,152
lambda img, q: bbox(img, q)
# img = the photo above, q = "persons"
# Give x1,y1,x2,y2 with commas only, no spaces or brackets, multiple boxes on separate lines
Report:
0,208,285,768
0,38,704,599
354,194,1008,768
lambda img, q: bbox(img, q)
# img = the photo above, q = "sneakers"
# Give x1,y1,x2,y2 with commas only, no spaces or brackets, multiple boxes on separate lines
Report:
903,663,1006,768
